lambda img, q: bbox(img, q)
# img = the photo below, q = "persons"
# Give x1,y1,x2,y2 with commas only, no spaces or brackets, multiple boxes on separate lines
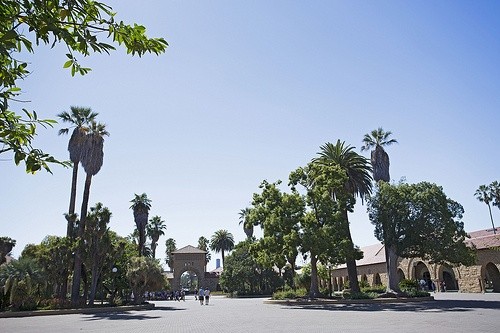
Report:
145,286,186,302
193,286,211,305
431,281,437,294
443,280,448,292
